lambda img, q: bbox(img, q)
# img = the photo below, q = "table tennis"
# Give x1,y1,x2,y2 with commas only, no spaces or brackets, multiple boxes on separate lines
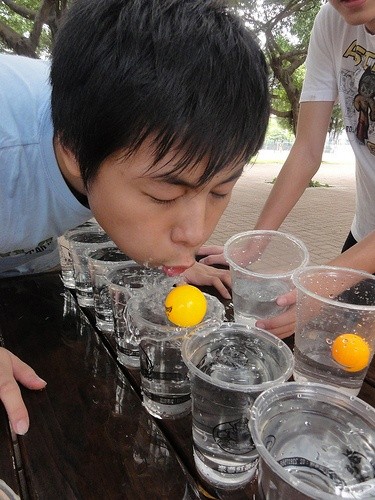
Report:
330,333,370,373
163,283,208,329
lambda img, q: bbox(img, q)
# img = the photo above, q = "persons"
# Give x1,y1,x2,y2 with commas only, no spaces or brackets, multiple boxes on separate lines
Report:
0,0,273,437
194,1,375,339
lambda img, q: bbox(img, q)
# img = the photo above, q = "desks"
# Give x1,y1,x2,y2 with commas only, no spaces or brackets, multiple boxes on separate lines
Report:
0,254,375,500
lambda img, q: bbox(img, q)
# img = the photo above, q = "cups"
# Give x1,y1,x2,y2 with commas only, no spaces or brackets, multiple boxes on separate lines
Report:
105,265,171,370
292,265,375,396
84,243,139,335
181,322,292,491
64,227,114,305
57,221,98,290
221,230,310,339
252,382,375,500
129,290,225,420
